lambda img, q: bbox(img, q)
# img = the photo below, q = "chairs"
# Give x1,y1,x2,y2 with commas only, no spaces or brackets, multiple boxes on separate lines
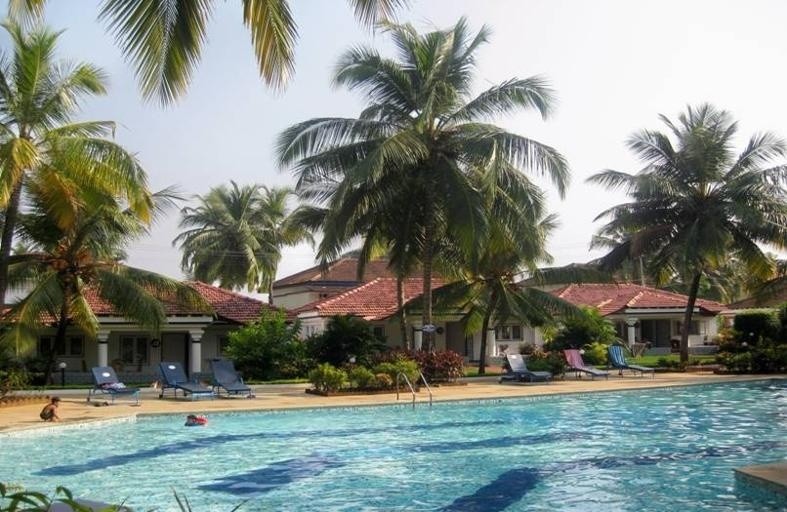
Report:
498,353,552,385
158,358,251,401
86,367,140,407
560,346,655,381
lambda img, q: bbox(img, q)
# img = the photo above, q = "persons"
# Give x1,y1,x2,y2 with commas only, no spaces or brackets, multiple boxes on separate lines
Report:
184,414,197,426
39,396,61,422
